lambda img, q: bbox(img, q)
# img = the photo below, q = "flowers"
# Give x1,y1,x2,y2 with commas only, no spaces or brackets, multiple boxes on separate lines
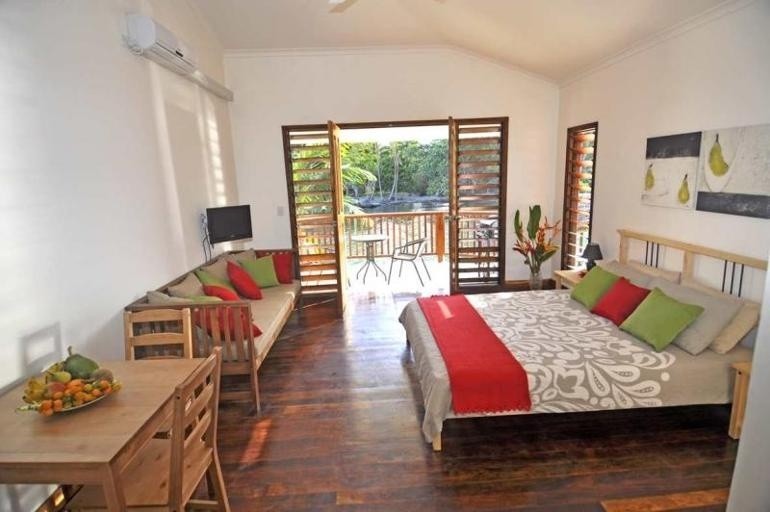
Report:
512,206,561,272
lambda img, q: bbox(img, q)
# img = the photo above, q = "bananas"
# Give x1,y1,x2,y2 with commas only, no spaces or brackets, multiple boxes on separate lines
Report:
23,362,62,404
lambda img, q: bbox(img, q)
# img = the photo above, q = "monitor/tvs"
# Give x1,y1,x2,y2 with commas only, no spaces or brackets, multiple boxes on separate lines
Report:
205,204,252,244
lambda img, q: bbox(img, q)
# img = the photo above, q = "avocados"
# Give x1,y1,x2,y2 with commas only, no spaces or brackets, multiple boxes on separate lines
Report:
63,347,99,381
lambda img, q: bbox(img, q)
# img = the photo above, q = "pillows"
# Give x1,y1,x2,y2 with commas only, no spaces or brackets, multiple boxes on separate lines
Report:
571,259,760,356
147,248,293,341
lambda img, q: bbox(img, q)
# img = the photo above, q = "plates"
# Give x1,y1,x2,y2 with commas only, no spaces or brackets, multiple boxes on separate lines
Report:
59,386,110,413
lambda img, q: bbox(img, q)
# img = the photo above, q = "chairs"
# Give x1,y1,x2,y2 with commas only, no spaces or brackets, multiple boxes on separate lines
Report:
64,347,231,512
388,238,431,287
123,308,216,502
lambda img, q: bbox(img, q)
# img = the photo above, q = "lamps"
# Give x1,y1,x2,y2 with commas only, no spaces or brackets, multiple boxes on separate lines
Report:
581,243,603,271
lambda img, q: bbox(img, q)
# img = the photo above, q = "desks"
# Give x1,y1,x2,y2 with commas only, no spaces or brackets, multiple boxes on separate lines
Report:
0,358,215,512
351,234,387,284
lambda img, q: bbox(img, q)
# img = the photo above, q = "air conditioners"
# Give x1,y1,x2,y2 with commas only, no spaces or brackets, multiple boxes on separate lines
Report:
125,15,200,76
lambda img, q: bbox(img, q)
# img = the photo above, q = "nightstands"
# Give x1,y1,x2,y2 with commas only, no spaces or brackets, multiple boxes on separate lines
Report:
553,271,590,290
728,361,751,440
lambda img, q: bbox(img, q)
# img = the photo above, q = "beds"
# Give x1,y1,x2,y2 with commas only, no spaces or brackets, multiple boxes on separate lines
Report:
399,229,770,451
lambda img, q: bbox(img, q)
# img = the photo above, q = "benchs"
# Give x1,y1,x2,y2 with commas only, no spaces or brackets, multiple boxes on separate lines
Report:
124,249,302,414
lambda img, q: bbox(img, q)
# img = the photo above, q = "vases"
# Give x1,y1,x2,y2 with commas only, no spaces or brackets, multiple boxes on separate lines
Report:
528,271,543,290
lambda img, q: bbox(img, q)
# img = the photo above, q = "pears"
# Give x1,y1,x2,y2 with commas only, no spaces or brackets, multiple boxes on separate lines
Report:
678,174,689,203
709,134,728,175
645,164,654,189
47,371,72,383
